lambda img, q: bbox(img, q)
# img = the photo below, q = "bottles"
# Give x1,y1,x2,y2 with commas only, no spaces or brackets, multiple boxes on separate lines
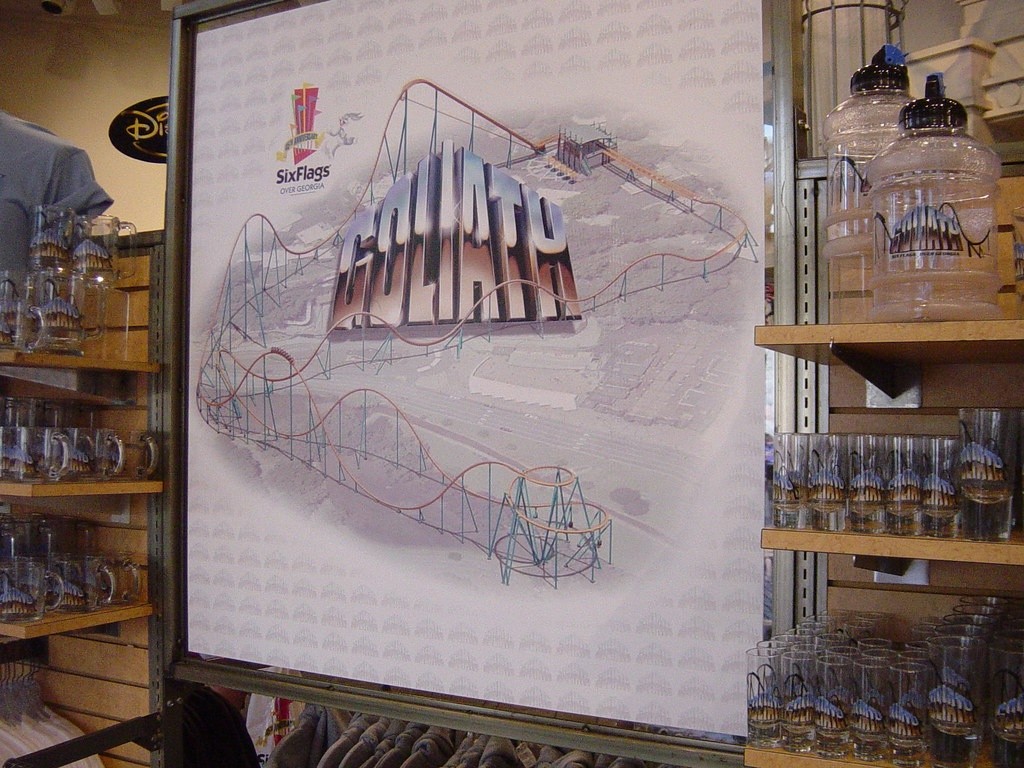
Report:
819,40,916,260
863,73,1000,320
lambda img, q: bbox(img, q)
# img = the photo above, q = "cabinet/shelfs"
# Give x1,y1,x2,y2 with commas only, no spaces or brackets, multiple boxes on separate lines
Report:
0,230,164,768
737,139,1024,768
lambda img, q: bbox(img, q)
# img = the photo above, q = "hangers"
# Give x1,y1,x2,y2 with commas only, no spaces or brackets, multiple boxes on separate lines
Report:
0,638,50,727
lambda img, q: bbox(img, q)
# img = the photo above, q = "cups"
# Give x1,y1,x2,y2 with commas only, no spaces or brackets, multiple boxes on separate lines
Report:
0,511,144,625
770,406,1024,544
745,593,1024,768
0,395,160,485
0,204,140,360
